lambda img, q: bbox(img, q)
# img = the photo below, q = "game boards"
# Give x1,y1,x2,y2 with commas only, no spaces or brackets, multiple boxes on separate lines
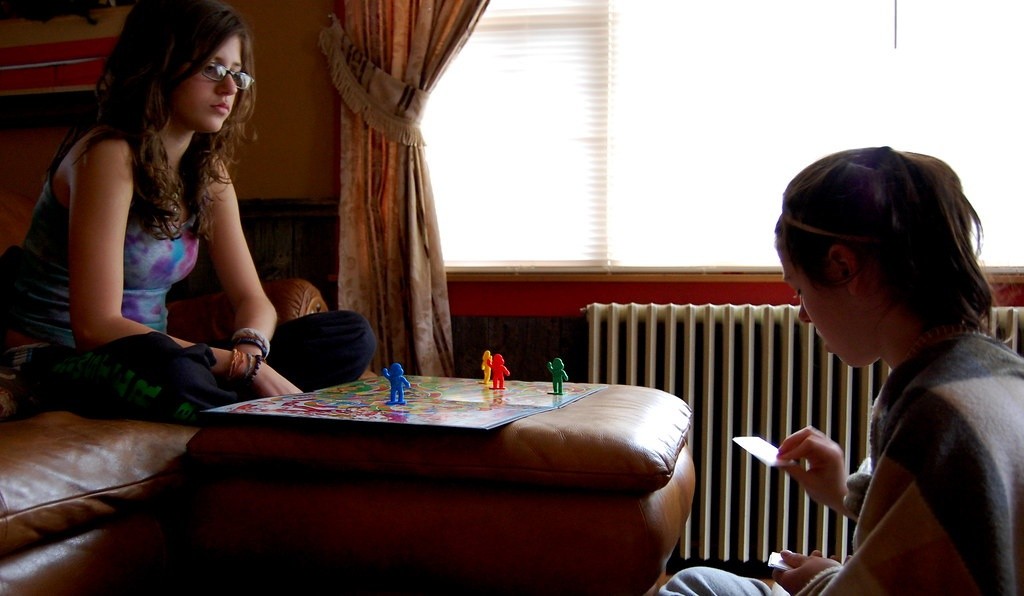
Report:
198,372,610,433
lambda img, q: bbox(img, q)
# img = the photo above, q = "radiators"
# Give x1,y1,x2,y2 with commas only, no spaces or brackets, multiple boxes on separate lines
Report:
580,302,1024,564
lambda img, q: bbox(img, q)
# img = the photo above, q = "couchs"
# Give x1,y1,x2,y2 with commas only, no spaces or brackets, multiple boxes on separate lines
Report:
0,191,697,596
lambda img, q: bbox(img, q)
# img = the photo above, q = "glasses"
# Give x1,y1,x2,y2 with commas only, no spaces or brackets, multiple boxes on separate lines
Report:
202,62,255,90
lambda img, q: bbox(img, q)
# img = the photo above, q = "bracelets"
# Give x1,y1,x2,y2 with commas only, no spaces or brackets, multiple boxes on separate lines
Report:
232,327,270,360
229,337,267,361
227,348,263,387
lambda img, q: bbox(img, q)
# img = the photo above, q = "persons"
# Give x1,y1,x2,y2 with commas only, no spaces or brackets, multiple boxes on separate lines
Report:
481,350,492,383
547,358,569,392
487,354,510,388
383,363,411,402
654,144,1024,596
0,1,378,431
